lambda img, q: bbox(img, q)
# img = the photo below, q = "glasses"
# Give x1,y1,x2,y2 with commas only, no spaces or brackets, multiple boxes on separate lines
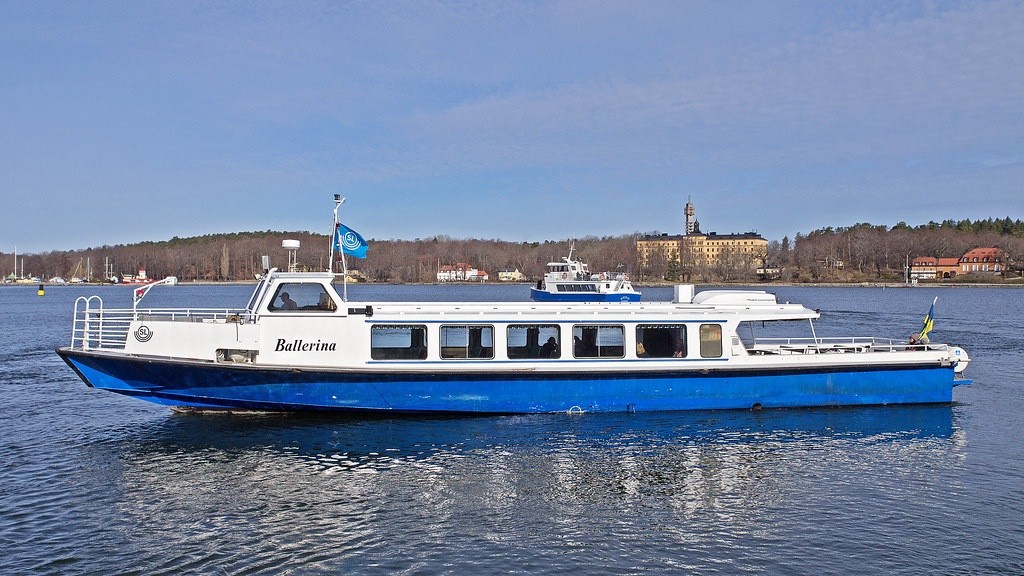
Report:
910,337,912,339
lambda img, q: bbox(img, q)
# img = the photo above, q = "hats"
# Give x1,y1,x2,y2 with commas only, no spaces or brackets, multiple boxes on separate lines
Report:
910,333,919,341
279,292,289,297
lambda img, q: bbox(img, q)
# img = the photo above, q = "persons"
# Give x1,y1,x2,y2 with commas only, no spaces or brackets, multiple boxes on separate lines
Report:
538,337,558,359
673,344,682,358
279,292,297,310
906,333,932,350
574,336,585,357
637,341,648,358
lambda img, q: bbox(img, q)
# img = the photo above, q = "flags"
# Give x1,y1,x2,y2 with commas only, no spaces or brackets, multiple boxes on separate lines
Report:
918,302,933,341
333,221,368,259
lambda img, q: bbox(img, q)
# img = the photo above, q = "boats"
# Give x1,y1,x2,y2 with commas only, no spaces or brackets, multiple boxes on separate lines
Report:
529,239,642,302
57,194,972,417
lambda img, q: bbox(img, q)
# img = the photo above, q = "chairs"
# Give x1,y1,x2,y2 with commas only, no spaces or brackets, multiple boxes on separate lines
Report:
405,344,427,360
470,345,488,358
522,345,560,360
